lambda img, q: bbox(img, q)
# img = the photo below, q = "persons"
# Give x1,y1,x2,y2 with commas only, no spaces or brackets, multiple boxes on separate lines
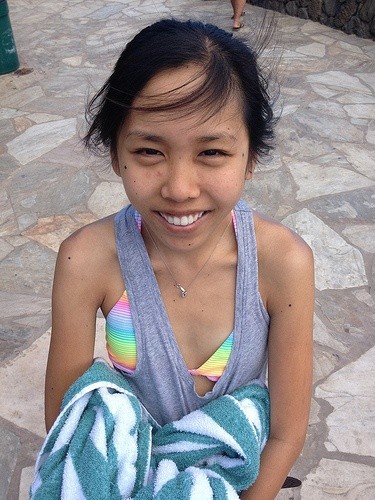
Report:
231,0,247,31
44,18,315,500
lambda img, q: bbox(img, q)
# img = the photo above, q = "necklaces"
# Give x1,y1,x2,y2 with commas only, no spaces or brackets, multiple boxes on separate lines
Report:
142,216,234,297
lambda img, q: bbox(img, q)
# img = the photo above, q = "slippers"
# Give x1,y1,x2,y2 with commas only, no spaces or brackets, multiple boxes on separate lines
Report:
231,11,245,19
232,22,245,31
